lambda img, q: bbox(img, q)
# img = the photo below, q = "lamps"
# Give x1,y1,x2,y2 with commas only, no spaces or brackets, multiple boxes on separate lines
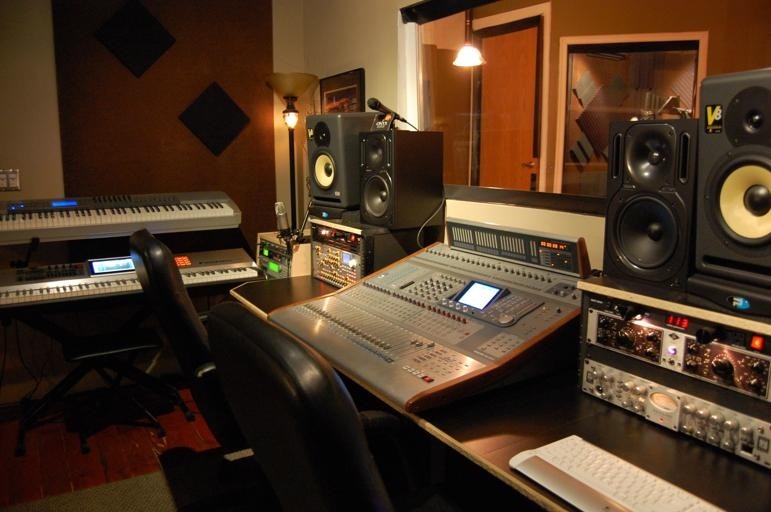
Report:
265,72,318,230
453,9,485,68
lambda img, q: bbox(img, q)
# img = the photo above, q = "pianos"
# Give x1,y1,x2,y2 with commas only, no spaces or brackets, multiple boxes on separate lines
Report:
0,191,242,246
0,248,258,308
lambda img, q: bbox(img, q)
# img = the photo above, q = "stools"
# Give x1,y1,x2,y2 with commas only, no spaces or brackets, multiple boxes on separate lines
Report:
66,329,163,454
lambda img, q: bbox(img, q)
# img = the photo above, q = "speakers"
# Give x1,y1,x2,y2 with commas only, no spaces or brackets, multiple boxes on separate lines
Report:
359,130,444,229
305,110,375,210
603,118,699,296
695,66,771,289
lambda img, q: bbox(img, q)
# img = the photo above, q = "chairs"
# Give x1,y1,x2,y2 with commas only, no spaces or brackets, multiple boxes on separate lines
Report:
207,300,416,510
129,229,222,392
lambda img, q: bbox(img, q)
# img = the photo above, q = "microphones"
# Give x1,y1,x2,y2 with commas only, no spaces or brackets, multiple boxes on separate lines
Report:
365,97,407,126
274,201,294,242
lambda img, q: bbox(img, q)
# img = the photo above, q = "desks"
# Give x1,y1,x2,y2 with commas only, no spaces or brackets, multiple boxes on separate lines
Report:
229,276,771,511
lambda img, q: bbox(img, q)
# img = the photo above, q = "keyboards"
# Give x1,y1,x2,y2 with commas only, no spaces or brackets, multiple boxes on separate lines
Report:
508,435,731,512
